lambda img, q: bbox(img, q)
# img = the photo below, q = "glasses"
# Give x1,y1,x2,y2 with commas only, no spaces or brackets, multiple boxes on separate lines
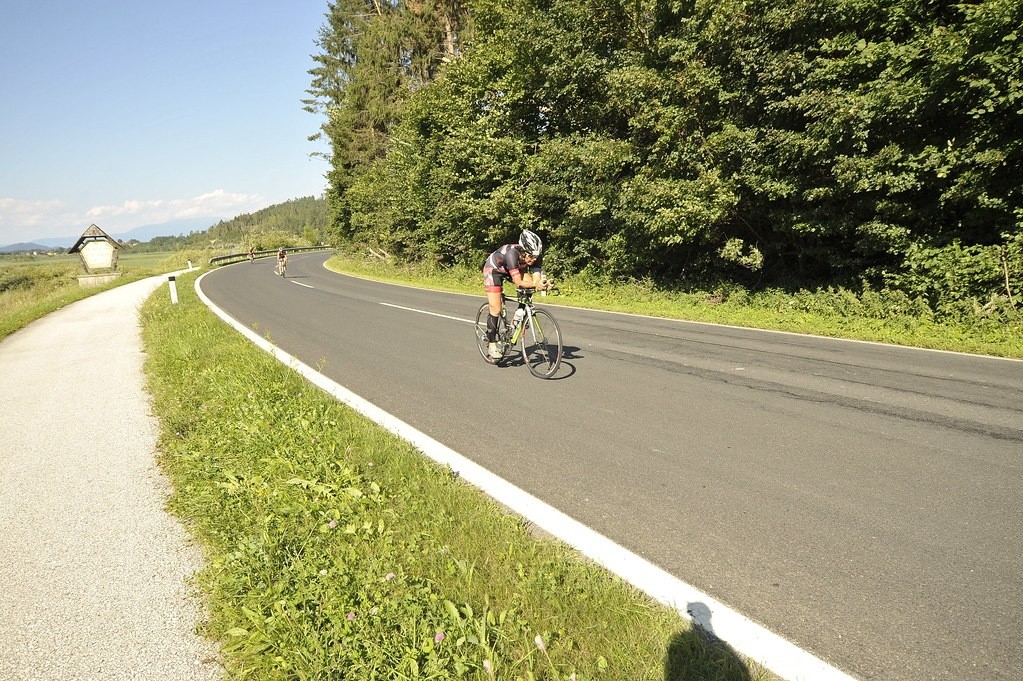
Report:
525,252,539,258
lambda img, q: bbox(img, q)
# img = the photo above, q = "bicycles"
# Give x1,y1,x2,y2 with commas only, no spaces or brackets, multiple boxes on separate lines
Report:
474,279,563,379
277,259,288,278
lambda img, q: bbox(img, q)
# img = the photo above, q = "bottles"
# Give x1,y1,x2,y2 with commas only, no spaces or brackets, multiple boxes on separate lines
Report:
510,308,524,329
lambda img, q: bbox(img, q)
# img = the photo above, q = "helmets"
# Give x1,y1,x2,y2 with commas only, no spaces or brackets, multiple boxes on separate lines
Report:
519,229,542,255
278,247,283,252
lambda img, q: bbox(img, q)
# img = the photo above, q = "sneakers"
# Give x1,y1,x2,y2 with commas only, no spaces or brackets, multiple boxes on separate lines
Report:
488,344,503,358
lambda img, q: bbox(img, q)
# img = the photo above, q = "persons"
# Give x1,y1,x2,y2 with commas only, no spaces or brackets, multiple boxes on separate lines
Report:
483,228,555,358
277,247,288,275
249,245,257,260
